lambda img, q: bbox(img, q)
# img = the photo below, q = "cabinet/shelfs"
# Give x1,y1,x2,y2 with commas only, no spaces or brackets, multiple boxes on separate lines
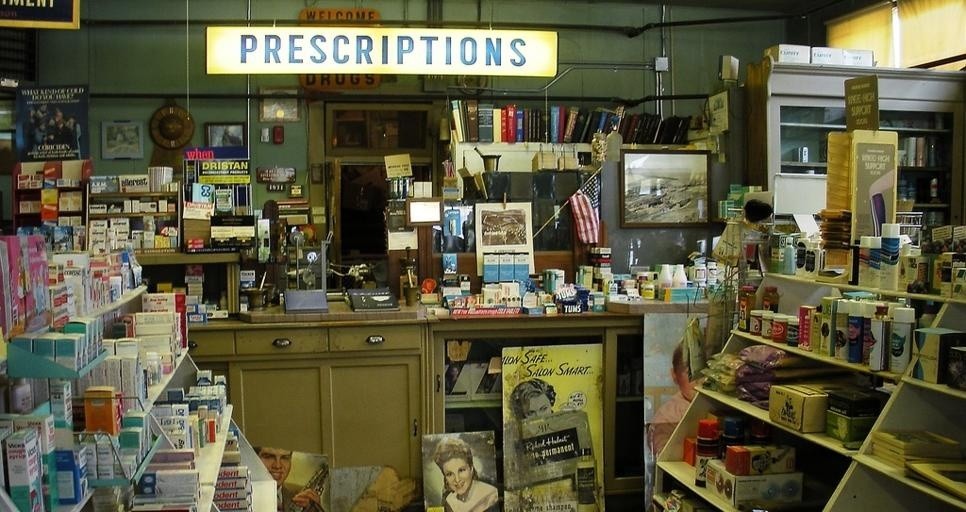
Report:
187,326,236,428
87,178,244,317
1,318,198,512
13,158,89,238
52,251,277,511
430,304,608,496
652,275,966,512
432,139,718,283
235,307,429,511
822,131,897,283
744,61,960,234
607,310,644,498
385,176,418,297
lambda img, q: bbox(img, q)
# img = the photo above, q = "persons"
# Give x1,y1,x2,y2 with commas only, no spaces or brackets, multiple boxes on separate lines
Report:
649,340,704,456
253,448,326,512
508,377,558,423
434,436,503,512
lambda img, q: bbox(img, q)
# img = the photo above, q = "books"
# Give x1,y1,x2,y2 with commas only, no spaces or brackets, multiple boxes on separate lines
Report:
872,426,962,473
448,95,693,146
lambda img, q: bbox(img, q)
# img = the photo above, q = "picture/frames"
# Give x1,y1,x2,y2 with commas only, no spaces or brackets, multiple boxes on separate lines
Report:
259,88,300,123
406,198,444,226
204,122,246,146
621,147,713,229
643,312,711,511
102,119,144,160
0,0,81,31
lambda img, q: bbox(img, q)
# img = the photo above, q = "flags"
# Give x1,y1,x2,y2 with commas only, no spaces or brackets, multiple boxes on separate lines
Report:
570,175,602,244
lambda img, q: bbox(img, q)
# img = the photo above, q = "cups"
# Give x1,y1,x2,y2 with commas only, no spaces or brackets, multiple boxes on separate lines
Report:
540,142,558,171
483,155,499,172
561,143,584,170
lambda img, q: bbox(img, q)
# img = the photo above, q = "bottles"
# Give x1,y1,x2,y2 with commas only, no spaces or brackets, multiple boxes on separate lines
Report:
917,137,927,167
750,310,763,336
11,379,32,415
919,305,937,327
859,236,870,287
639,276,648,296
220,290,227,310
908,137,916,166
863,303,876,366
721,415,746,459
641,281,647,298
659,265,672,301
652,274,658,298
927,135,937,168
696,420,721,488
787,319,799,346
762,286,779,312
870,304,889,371
752,422,770,445
880,224,900,291
898,242,911,292
930,173,939,203
848,301,863,364
898,170,907,199
805,242,820,279
694,261,706,281
772,316,788,342
644,280,655,299
890,308,916,374
738,286,756,332
796,232,809,277
762,314,774,339
782,238,796,275
707,261,716,279
848,239,860,286
868,237,881,288
835,299,851,360
812,305,823,351
673,264,687,287
906,182,916,203
